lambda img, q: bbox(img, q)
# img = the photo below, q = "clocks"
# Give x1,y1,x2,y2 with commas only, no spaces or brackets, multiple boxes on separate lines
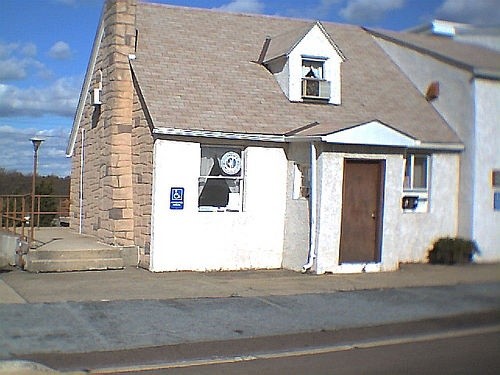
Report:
221,151,243,175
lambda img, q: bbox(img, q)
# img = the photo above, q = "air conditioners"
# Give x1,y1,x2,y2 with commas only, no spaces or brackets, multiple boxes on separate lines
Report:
90,88,103,105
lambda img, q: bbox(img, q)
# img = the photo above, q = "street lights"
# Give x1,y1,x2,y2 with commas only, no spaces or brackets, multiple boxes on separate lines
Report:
28,136,45,240
